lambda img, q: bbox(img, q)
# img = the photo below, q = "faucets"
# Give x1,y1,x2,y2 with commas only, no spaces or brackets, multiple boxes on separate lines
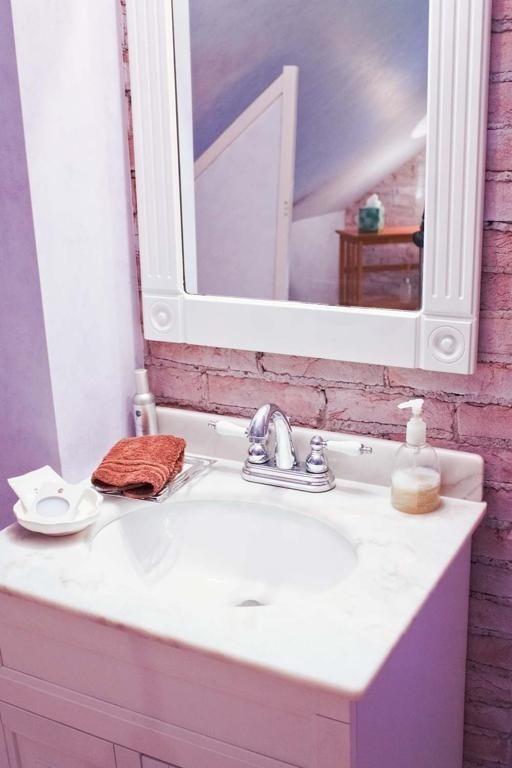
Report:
247,401,298,470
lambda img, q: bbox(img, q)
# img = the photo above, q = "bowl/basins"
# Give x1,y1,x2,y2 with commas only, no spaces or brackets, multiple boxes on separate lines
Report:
12,485,104,537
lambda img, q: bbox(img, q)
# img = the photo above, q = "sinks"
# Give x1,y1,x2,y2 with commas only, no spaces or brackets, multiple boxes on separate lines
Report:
85,494,355,606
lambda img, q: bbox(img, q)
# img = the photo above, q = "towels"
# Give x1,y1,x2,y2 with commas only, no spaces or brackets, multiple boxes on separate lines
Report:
92,435,185,499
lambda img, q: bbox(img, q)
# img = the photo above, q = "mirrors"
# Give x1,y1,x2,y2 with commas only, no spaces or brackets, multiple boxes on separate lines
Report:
124,0,496,376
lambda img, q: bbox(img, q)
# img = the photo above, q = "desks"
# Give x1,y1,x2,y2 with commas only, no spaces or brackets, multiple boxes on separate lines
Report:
336,227,423,311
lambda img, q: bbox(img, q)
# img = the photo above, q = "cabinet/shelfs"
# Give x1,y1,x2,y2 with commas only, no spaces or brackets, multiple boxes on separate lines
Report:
0,664,300,767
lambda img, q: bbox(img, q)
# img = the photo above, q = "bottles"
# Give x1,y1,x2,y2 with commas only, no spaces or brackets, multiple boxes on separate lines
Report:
131,369,159,437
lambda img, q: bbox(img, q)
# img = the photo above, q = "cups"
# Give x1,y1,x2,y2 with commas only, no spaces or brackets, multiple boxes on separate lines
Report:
358,207,379,234
